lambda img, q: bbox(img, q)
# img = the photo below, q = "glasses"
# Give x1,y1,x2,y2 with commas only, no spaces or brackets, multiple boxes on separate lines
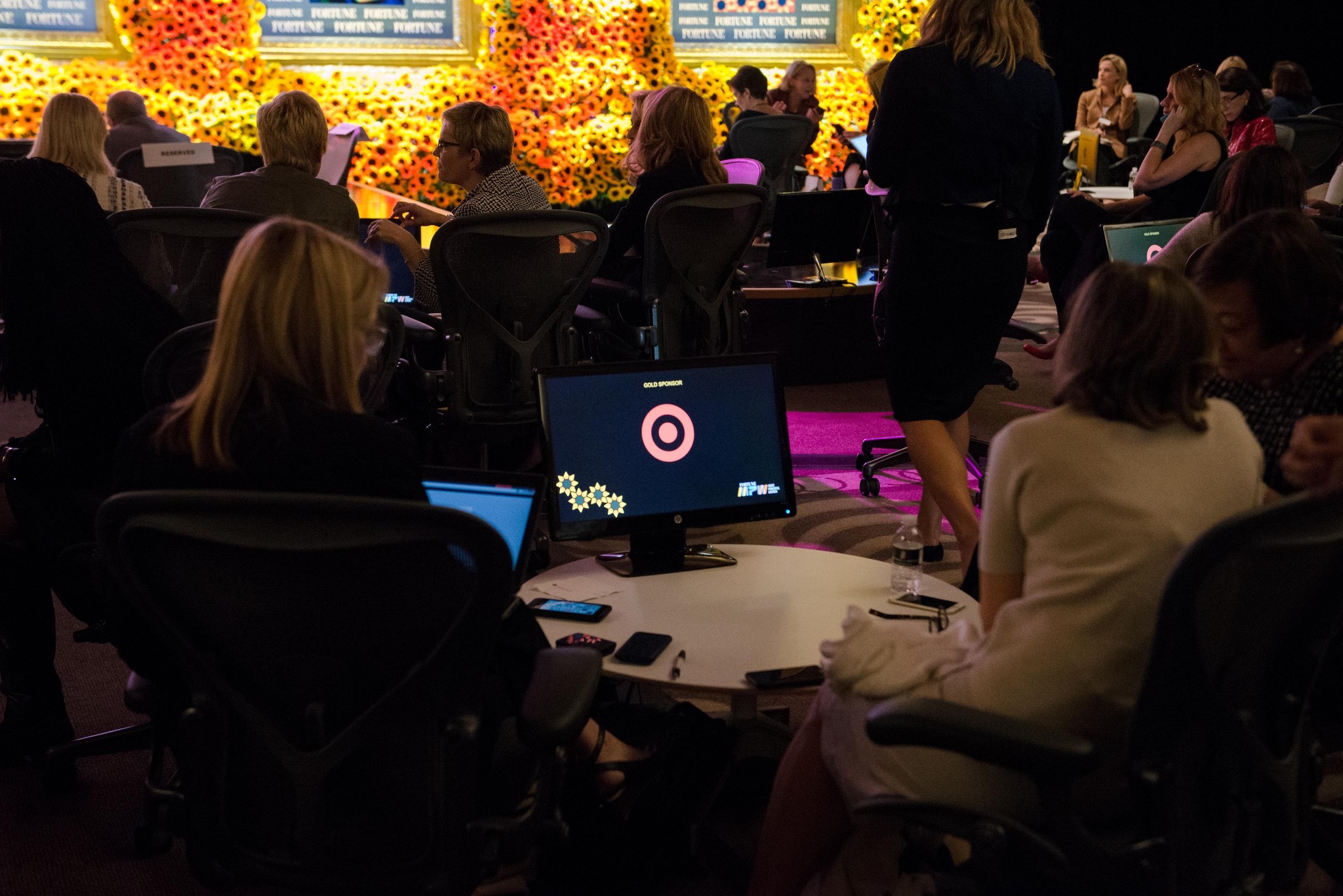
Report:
1189,62,1205,99
1220,93,1241,107
352,321,390,357
438,138,461,152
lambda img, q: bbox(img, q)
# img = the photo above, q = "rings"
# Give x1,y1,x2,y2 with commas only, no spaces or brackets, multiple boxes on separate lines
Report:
370,229,374,235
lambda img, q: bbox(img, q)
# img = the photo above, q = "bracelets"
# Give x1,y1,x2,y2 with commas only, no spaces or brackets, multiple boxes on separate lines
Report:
404,249,423,263
447,214,454,222
1150,141,1166,153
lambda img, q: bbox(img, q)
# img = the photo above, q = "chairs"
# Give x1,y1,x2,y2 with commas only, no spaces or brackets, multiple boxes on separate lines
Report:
849,481,1342,896
1057,90,1342,235
84,487,622,895
0,126,886,816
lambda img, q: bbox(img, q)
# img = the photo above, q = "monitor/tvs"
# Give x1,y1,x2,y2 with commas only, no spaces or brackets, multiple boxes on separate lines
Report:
532,358,796,543
358,217,420,308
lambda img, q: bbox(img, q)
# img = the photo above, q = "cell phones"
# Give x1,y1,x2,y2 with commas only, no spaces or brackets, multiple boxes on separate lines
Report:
527,597,613,624
1160,113,1171,124
614,630,673,665
888,591,964,616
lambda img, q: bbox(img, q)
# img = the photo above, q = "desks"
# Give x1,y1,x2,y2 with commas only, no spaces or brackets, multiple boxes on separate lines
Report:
516,539,990,787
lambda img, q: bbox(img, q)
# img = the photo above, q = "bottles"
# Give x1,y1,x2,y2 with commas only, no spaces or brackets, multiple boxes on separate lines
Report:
892,514,923,594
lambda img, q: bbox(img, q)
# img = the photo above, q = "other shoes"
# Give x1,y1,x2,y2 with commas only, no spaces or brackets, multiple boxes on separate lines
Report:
920,540,944,562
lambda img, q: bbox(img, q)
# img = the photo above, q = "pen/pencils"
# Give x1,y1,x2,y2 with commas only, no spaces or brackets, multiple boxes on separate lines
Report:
672,650,685,677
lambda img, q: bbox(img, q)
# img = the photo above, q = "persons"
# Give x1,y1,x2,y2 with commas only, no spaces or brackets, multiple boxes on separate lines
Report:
754,259,1277,896
567,86,729,342
549,88,654,224
104,90,192,165
832,56,893,189
19,92,181,308
1192,206,1342,496
364,99,564,365
1068,52,1142,185
1262,59,1322,123
148,212,655,895
712,62,794,160
1214,67,1278,155
1143,143,1309,272
767,60,826,148
177,89,364,312
1024,64,1228,363
1,154,204,828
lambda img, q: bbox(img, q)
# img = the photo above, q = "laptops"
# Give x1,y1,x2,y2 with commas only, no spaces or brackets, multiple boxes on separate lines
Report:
418,470,545,593
847,133,868,161
1102,216,1196,263
